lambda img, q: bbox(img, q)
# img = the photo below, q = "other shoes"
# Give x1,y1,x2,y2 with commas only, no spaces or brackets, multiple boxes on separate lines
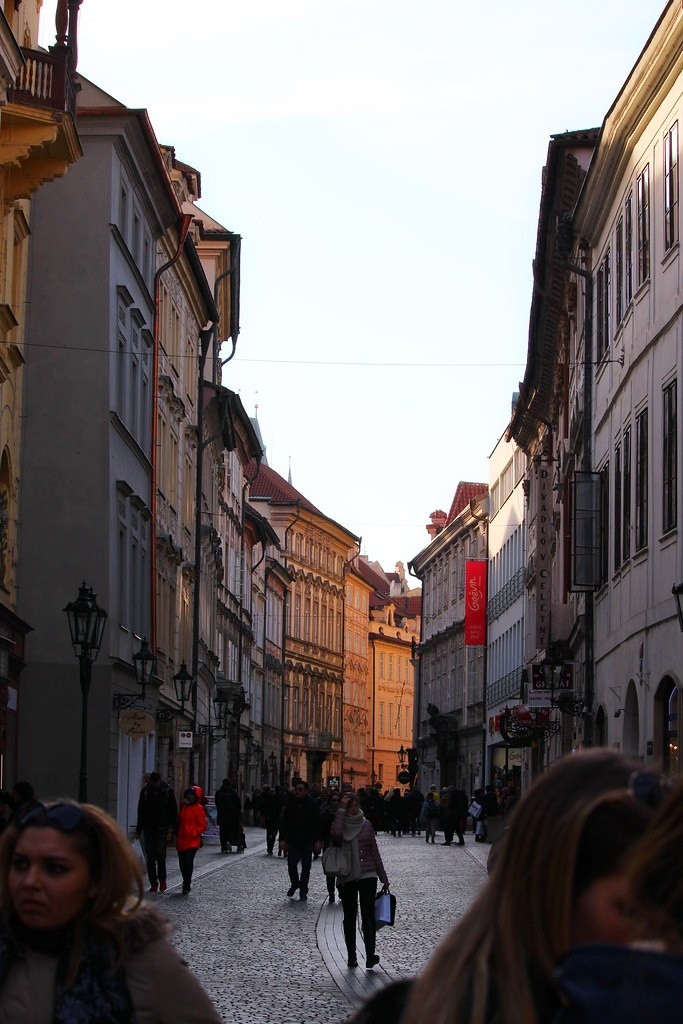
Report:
454,840,464,846
328,890,335,902
287,885,298,896
182,883,191,894
300,892,307,900
365,956,379,968
159,880,166,892
150,882,158,892
348,960,358,966
440,841,451,846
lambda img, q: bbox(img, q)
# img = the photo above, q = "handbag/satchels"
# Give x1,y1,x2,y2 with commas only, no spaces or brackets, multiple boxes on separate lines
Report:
467,801,482,818
374,886,396,930
475,821,483,834
322,836,351,877
131,839,146,865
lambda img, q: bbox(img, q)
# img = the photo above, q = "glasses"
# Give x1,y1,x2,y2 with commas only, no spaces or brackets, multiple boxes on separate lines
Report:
15,800,102,849
629,769,673,809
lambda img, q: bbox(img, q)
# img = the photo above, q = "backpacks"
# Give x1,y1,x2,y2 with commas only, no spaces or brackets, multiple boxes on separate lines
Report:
424,800,437,819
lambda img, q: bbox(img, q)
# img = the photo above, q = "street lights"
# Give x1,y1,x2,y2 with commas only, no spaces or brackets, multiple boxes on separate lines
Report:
63,578,109,805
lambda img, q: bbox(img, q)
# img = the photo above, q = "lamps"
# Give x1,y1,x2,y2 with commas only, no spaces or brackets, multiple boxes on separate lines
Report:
284,757,293,775
112,636,155,713
209,709,232,745
613,709,639,717
366,771,377,788
398,746,412,768
345,765,355,784
247,741,264,772
540,647,586,721
155,659,194,723
198,687,229,736
238,730,255,764
263,751,276,775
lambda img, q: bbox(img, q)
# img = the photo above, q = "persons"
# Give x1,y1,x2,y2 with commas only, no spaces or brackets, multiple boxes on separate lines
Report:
352,751,683,1024
1,780,40,829
244,783,514,859
135,773,178,891
214,779,241,852
279,781,323,900
0,800,224,1024
179,785,206,893
330,792,389,968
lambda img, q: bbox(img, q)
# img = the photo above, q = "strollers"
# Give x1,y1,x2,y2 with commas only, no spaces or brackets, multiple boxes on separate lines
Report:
226,823,245,853
